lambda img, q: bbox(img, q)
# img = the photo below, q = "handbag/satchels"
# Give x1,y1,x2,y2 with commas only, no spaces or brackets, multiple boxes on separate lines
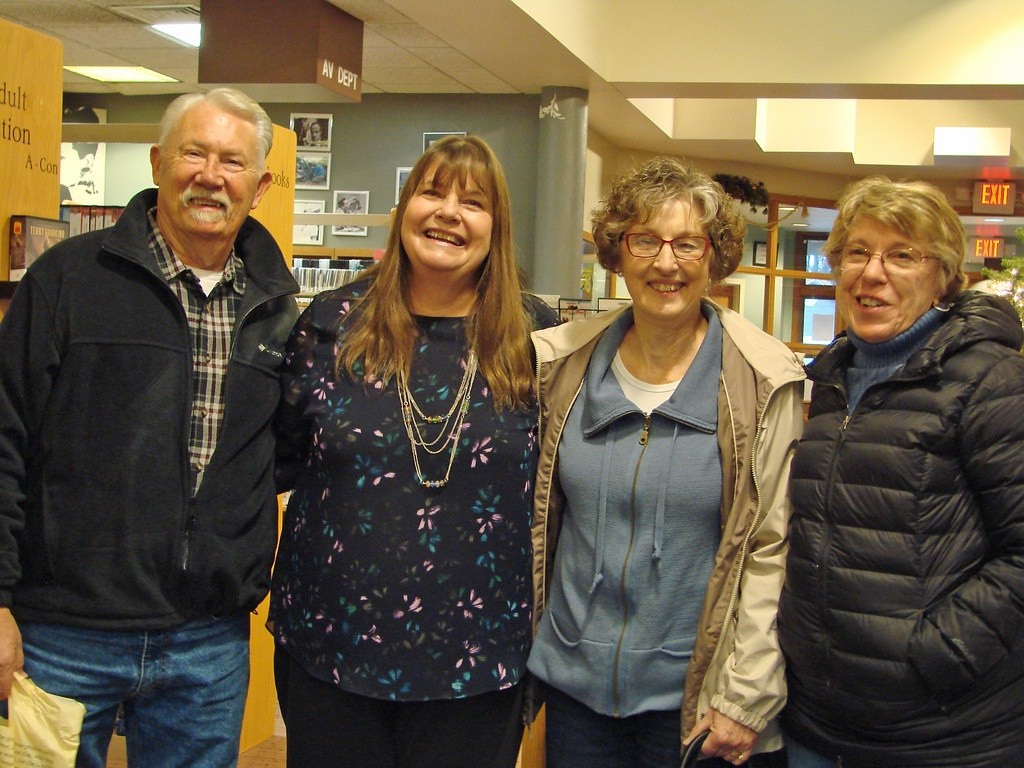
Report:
1,666,86,767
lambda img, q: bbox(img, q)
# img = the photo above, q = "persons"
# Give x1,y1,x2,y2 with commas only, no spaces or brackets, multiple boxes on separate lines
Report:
519,158,809,768
273,135,568,767
0,86,305,768
772,177,1024,768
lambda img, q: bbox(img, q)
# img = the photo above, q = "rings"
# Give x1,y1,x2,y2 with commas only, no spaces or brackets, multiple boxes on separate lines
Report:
730,750,743,759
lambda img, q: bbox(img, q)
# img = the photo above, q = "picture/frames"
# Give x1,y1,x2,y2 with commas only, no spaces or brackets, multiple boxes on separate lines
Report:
331,190,370,238
296,150,332,191
753,241,780,268
290,111,333,152
422,131,469,156
579,263,594,301
598,297,631,314
61,91,107,208
558,298,594,324
293,199,326,246
395,166,414,206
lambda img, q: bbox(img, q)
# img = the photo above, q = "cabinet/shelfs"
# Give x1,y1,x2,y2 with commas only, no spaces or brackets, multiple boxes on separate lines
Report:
291,245,388,315
0,14,64,330
60,117,297,278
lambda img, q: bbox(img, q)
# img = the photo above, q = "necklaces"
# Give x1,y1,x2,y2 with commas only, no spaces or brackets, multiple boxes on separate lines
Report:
391,309,482,491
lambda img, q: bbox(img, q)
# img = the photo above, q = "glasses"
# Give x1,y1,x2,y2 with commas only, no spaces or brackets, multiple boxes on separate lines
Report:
830,240,941,276
620,229,715,261
